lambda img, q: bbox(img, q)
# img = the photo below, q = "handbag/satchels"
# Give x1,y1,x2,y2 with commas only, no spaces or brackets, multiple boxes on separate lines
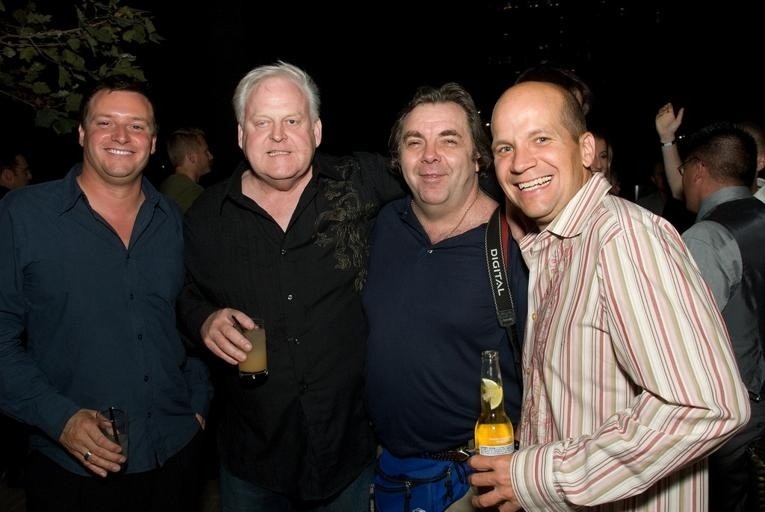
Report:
372,448,478,512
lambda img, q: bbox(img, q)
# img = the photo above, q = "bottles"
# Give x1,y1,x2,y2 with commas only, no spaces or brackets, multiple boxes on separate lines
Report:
474,348,515,511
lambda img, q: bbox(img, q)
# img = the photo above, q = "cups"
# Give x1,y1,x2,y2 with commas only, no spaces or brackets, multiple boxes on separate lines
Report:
95,406,129,469
234,318,269,380
